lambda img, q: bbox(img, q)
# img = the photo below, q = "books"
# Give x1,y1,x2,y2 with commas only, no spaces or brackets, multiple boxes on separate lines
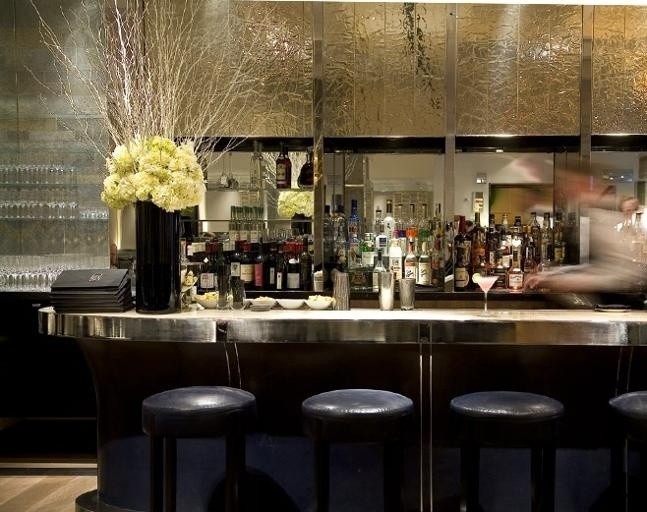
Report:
48,269,134,313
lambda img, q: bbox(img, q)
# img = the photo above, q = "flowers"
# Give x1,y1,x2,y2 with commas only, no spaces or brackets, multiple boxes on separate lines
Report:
99,136,205,213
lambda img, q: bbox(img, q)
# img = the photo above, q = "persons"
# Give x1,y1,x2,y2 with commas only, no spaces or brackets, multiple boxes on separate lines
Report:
523,198,647,295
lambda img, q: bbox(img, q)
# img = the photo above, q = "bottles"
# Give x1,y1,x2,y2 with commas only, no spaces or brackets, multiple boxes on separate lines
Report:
180,206,312,291
323,199,567,291
619,210,647,264
217,140,313,191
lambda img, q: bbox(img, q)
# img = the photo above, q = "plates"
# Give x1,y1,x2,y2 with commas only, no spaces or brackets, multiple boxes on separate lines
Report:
595,308,632,313
194,293,332,310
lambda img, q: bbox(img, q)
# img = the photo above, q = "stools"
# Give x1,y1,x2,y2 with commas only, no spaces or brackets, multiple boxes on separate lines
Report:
448,387,563,510
139,385,262,511
302,386,413,512
607,388,647,510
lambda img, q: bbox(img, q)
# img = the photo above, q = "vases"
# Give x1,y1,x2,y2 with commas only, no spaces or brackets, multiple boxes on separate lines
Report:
131,201,179,315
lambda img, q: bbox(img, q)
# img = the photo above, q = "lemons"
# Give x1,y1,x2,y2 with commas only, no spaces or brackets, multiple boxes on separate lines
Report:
472,273,480,284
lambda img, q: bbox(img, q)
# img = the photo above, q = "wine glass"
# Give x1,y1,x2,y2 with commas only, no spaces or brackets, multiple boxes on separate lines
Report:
474,276,500,317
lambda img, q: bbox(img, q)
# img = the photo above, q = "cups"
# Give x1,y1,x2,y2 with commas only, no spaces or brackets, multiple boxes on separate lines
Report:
379,272,395,312
333,272,350,311
399,278,416,310
